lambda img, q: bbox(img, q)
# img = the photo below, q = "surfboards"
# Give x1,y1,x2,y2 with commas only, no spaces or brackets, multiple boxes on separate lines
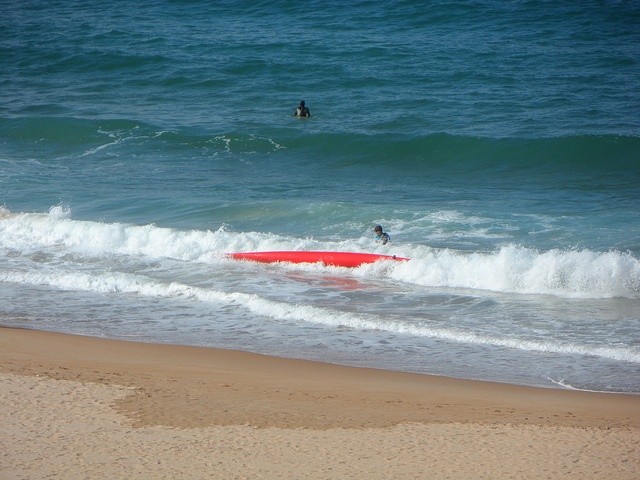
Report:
225,252,412,268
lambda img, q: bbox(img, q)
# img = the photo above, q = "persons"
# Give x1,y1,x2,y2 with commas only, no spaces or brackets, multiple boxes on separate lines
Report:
293,100,311,117
374,225,390,245
